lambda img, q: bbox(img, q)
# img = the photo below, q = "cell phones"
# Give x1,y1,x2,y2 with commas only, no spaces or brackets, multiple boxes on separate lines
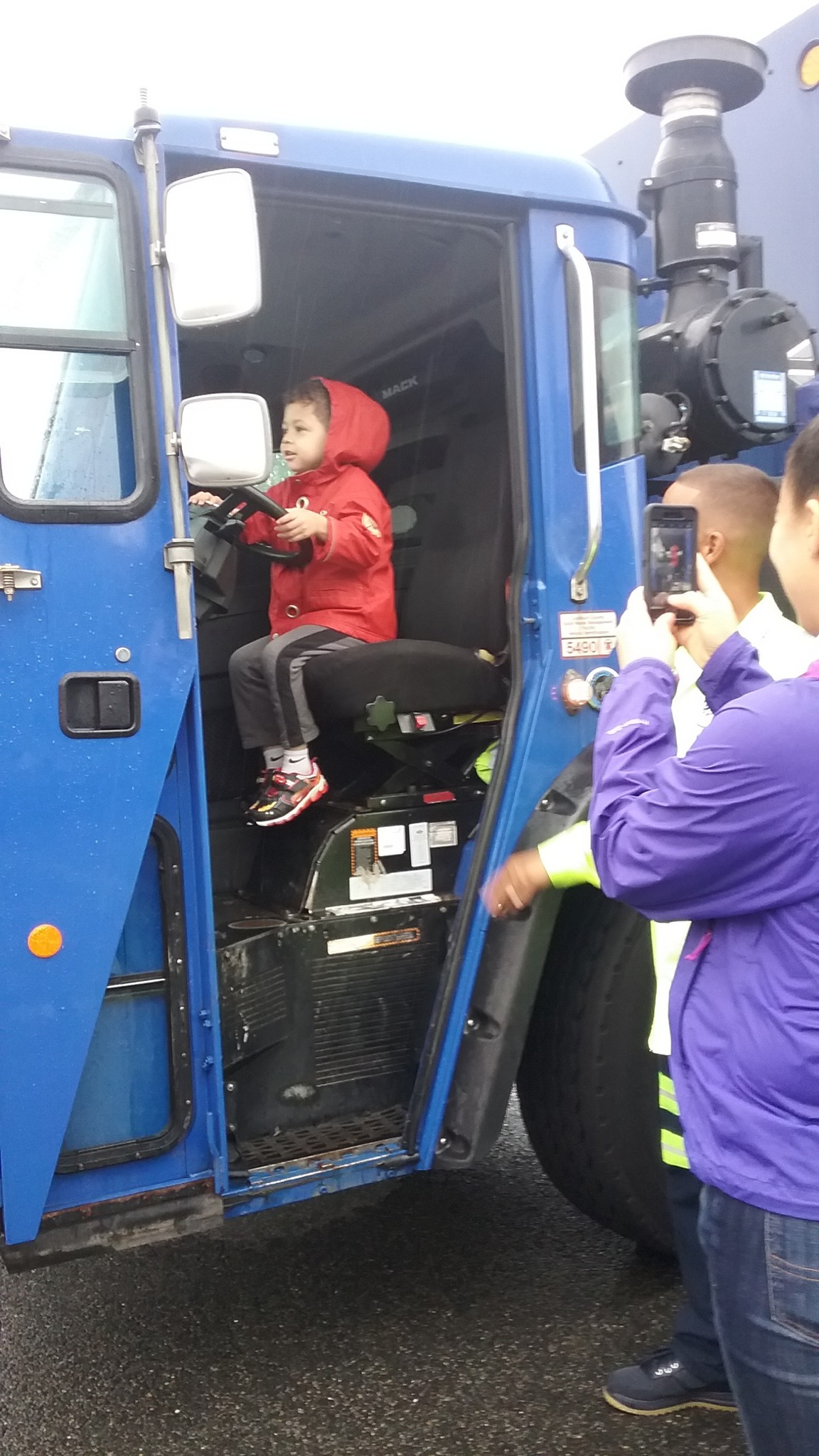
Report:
641,504,699,625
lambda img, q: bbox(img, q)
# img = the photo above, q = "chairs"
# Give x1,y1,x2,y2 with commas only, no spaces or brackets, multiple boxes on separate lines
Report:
303,426,514,810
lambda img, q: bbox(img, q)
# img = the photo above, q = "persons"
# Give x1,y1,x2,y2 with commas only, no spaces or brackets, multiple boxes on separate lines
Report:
480,464,819,1419
186,378,396,827
590,411,819,1456
666,544,680,574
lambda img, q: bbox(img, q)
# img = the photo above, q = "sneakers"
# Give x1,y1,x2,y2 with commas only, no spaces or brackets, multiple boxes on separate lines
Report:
603,1345,739,1415
245,756,328,826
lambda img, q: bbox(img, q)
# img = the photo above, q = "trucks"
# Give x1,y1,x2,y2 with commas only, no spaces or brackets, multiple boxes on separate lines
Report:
0,8,817,1278
